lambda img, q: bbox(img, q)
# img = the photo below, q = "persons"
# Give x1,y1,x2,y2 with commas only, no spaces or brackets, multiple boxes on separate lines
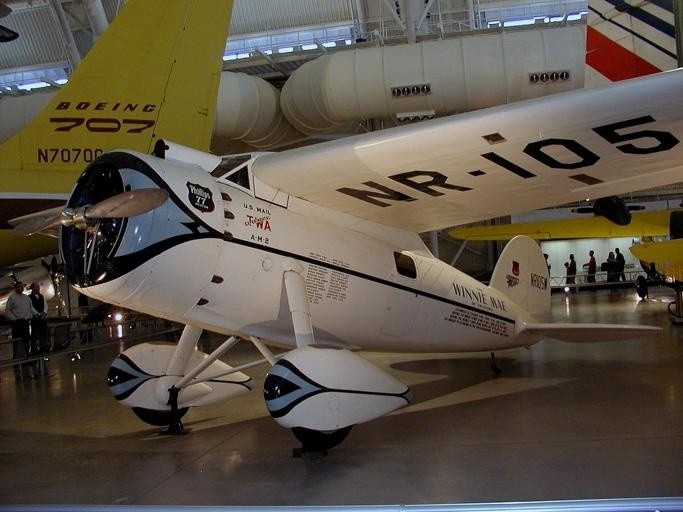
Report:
605,251,618,293
543,253,551,278
614,247,625,281
563,261,569,284
581,249,595,282
28,282,48,356
5,281,34,366
568,253,576,284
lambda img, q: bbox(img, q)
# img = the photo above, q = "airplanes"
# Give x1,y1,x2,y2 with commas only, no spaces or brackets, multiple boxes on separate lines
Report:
0,65,683,464
445,201,682,327
0,1,244,222
